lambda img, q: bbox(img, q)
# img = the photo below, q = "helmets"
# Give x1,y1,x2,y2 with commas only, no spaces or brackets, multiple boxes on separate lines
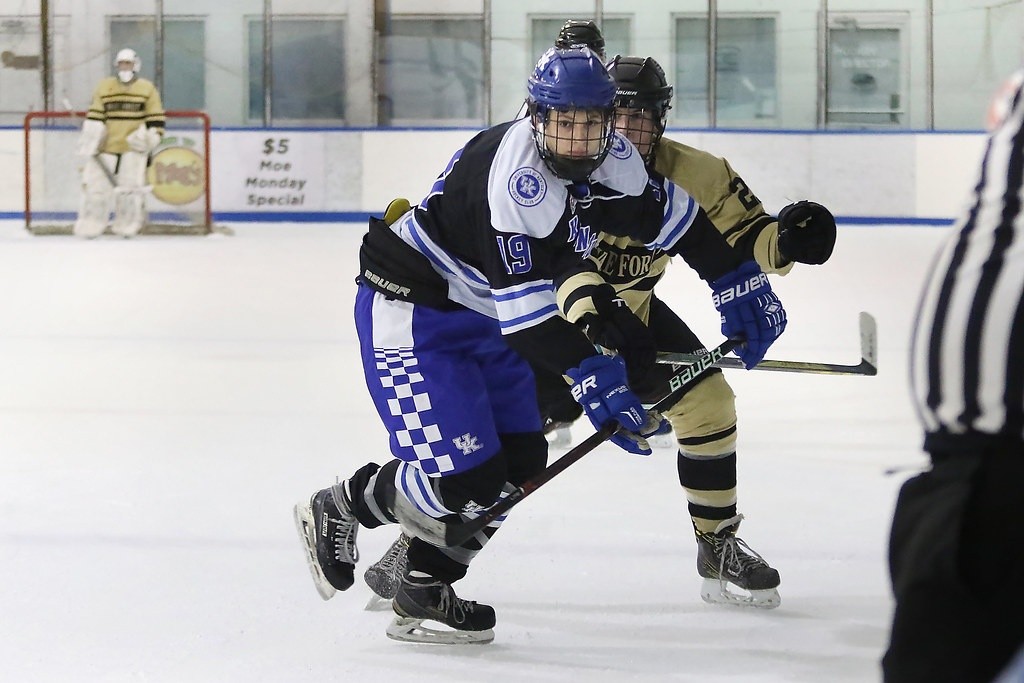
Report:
526,47,618,180
606,54,673,164
554,20,607,65
113,48,141,83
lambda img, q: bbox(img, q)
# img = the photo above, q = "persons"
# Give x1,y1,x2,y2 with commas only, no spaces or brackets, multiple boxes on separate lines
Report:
74,49,165,237
361,19,837,610
881,81,1024,682
292,40,789,642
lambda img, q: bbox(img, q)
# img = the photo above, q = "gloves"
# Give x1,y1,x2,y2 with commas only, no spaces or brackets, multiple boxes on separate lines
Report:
562,354,672,456
777,200,837,266
573,283,657,379
709,261,787,370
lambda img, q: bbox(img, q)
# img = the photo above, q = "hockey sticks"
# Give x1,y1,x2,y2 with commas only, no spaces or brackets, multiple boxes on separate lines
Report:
379,330,750,552
655,308,881,379
61,95,156,198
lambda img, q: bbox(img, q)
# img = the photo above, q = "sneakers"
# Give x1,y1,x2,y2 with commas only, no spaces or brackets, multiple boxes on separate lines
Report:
691,513,781,608
363,531,411,609
386,572,496,644
294,480,359,601
